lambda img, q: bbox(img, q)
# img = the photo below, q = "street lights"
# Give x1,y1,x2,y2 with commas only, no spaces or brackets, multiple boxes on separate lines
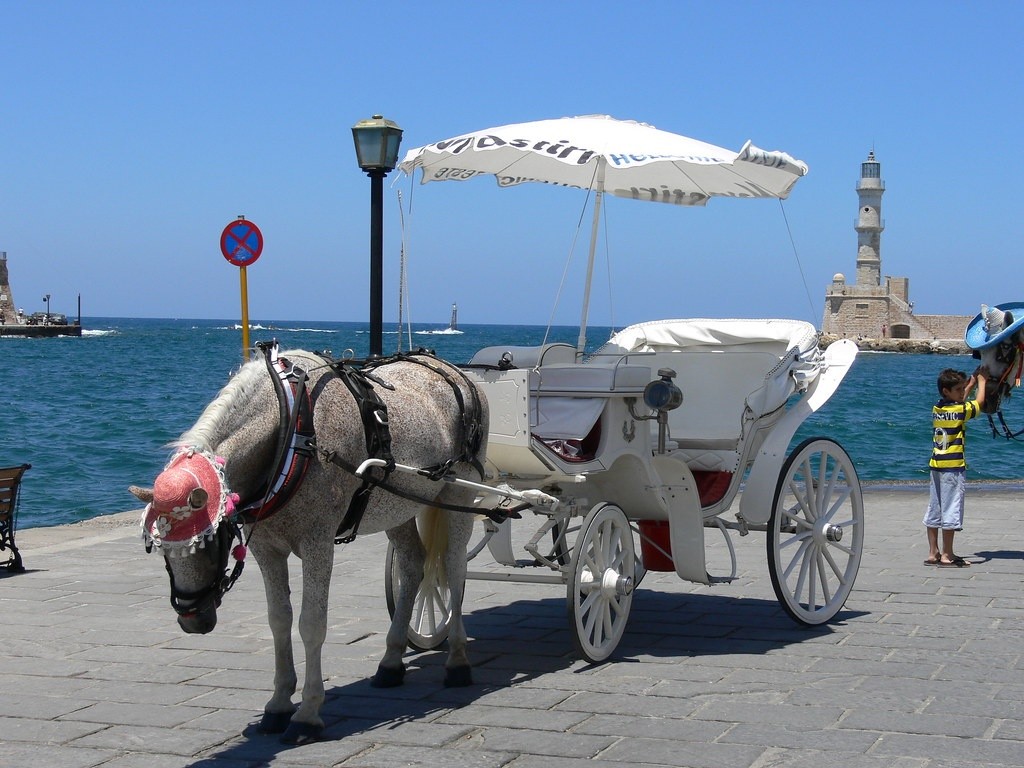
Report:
350,112,404,364
43,294,50,318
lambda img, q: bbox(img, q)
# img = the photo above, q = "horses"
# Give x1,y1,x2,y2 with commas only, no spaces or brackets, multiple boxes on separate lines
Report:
127,350,491,745
975,310,1024,415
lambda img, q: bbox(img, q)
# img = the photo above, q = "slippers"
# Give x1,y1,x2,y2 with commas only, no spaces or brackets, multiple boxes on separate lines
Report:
937,555,970,568
924,553,941,566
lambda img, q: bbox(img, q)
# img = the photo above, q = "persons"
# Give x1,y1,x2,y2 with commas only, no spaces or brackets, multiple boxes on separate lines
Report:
922,364,986,569
18,307,23,317
0,309,4,315
43,315,47,325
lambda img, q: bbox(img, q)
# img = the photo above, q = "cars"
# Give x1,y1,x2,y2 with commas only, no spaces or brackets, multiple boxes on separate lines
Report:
50,314,68,325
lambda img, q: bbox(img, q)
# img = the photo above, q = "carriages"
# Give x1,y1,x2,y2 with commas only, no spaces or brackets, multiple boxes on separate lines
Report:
129,114,866,745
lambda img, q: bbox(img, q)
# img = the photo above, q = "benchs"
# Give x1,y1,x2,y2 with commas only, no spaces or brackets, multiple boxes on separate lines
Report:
615,350,781,449
0,463,32,573
526,350,656,394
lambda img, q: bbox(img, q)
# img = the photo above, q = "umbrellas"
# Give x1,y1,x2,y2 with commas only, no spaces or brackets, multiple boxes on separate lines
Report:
397,116,808,364
